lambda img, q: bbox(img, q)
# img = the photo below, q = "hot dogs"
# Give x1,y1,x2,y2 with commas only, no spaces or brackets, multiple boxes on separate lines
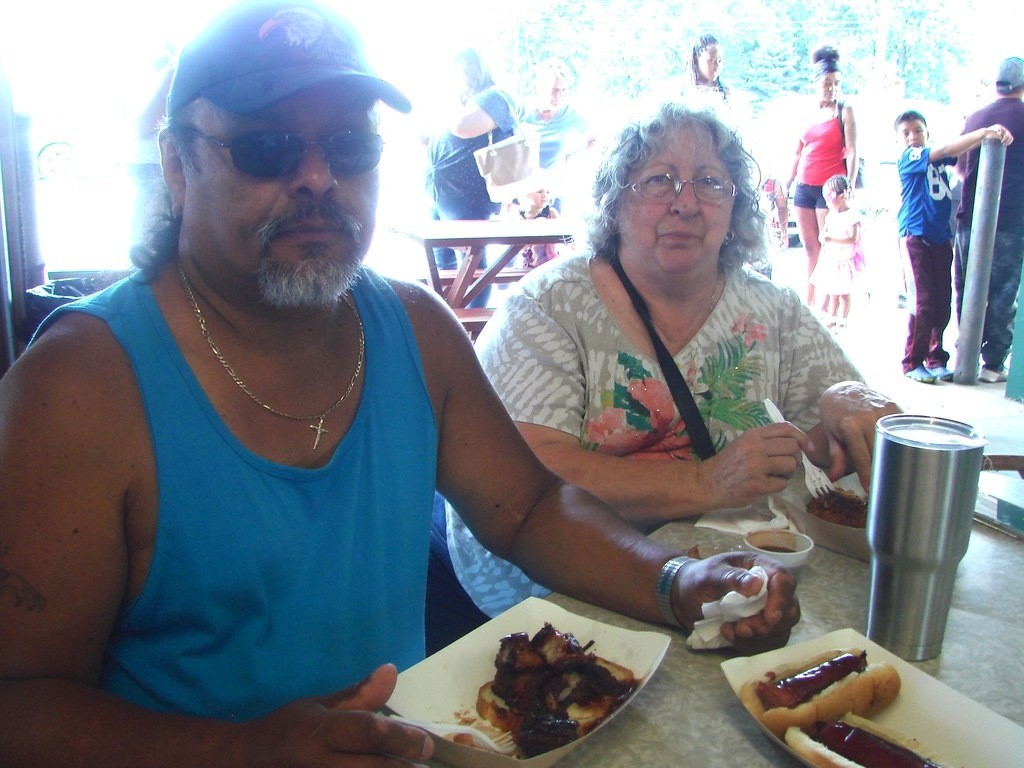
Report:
739,646,902,734
783,713,956,768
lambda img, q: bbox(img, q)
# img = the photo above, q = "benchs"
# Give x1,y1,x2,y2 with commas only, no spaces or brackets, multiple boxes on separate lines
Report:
452,310,494,330
437,269,534,283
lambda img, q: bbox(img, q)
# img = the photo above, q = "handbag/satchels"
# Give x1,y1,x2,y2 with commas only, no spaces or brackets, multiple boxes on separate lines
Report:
838,100,864,189
473,87,545,203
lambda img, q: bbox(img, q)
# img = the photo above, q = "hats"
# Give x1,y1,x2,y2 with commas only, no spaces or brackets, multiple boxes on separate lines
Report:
166,0,412,116
996,56,1024,92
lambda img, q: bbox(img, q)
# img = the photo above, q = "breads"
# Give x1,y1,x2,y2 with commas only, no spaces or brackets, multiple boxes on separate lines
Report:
805,491,867,527
476,653,636,738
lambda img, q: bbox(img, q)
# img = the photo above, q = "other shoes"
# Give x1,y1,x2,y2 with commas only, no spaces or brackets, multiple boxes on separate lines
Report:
977,362,1009,383
823,319,848,334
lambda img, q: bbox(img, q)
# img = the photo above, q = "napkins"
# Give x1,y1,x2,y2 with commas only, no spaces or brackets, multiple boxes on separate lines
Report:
686,565,768,650
694,491,809,534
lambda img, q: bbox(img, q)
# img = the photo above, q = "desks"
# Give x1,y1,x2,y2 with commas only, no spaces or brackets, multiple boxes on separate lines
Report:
394,219,575,310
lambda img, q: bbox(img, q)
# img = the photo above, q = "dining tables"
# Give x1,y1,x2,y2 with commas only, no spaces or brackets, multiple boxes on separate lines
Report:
542,463,1024,768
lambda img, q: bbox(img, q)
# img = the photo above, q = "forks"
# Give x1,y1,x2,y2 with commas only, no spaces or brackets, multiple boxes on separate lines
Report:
389,714,520,755
763,398,836,500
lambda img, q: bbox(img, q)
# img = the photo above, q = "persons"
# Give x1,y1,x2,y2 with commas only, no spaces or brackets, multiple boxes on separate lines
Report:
897,110,1014,385
0,0,801,768
785,46,869,338
401,43,591,347
672,33,789,290
955,56,1024,383
446,90,903,620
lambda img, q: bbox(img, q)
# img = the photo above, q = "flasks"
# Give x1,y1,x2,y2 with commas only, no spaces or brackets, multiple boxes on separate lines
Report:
866,413,985,663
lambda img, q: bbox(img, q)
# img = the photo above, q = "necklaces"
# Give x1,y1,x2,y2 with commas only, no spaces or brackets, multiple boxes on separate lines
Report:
176,256,366,451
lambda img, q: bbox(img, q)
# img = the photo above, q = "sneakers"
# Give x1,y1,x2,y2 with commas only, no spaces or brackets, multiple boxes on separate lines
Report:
905,365,955,383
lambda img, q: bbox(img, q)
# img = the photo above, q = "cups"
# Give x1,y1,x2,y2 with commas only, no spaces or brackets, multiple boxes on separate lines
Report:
743,527,814,580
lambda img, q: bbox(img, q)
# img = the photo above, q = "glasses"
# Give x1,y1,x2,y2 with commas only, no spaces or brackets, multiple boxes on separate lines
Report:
188,128,385,179
625,168,740,204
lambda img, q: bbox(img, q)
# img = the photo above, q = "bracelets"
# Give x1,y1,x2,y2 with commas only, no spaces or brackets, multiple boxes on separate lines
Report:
656,554,703,634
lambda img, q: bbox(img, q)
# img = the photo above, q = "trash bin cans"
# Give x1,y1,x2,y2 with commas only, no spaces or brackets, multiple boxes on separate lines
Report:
25,278,116,345
